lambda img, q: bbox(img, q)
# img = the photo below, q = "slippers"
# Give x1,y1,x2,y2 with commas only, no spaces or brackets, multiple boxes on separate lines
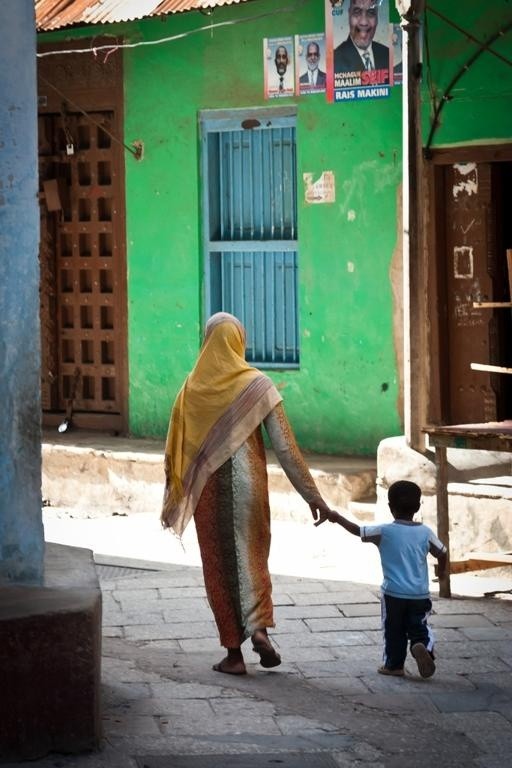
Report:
251,644,281,668
211,663,248,677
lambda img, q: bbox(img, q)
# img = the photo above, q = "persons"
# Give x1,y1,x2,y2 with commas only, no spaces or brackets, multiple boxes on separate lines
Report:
161,310,329,676
333,0,389,87
328,480,449,678
300,43,326,87
273,45,290,75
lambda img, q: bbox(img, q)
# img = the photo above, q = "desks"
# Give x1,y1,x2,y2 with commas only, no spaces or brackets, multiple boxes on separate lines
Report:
420,420,511,599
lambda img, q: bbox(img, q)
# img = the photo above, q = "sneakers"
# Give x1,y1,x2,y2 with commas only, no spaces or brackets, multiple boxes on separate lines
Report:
375,664,406,677
410,644,436,679
